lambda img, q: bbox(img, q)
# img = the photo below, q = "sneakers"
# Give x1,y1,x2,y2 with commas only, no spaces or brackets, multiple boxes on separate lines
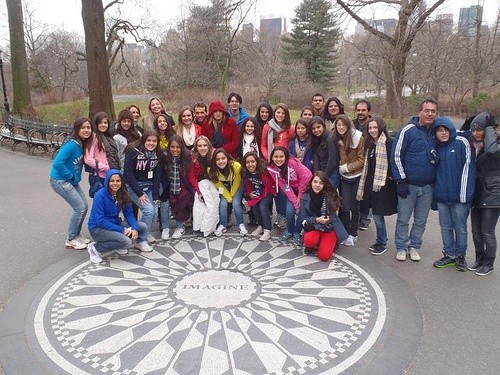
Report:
258,229,271,241
215,224,227,236
408,247,421,261
433,251,456,268
115,248,129,255
395,249,407,261
171,226,185,238
251,225,264,236
238,223,248,236
134,240,153,252
455,255,467,271
292,232,301,245
161,228,169,240
279,230,292,241
64,235,91,250
145,233,155,242
340,235,358,246
369,244,387,255
87,242,102,263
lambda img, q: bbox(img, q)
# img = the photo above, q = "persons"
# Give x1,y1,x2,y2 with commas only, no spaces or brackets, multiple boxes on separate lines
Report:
296,170,348,261
84,93,397,256
390,99,438,261
87,169,147,263
49,117,91,250
459,112,500,276
430,117,476,272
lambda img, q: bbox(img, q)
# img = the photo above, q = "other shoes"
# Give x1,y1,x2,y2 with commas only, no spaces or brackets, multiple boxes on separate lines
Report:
304,246,314,254
358,218,371,230
274,213,287,228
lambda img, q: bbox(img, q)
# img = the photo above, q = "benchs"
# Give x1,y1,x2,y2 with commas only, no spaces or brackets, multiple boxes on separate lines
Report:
0,115,73,159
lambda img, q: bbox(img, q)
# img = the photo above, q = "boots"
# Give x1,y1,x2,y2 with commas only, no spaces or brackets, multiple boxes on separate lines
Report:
476,256,495,275
467,252,486,270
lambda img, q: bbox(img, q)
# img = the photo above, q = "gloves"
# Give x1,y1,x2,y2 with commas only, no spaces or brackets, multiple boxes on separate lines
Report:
486,114,495,126
396,179,411,199
463,115,476,131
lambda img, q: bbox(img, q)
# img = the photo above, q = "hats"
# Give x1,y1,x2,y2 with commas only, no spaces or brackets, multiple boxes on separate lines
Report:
470,111,489,129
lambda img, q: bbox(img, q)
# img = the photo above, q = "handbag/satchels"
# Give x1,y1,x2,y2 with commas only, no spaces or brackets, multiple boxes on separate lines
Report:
88,159,103,198
